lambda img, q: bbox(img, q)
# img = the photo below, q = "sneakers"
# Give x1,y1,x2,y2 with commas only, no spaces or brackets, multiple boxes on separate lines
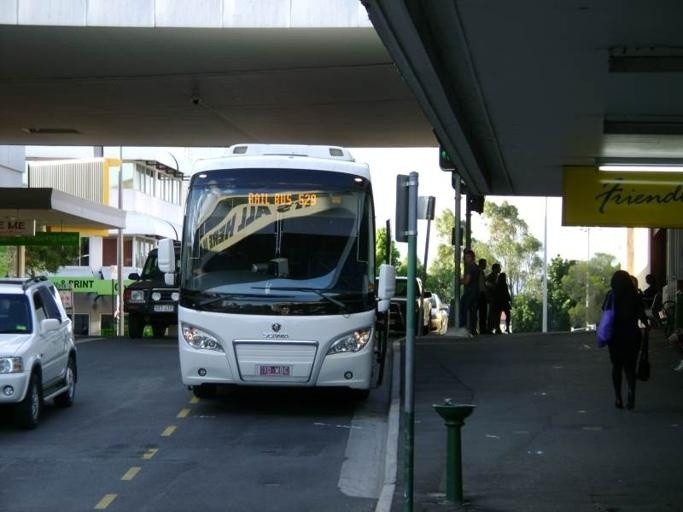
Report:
614,399,635,411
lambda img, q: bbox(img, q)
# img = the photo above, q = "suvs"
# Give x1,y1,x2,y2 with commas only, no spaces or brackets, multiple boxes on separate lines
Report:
374,274,452,334
0,274,78,424
121,236,184,340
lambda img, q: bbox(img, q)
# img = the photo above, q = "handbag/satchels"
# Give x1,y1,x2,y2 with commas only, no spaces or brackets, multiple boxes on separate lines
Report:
594,311,614,350
637,355,652,383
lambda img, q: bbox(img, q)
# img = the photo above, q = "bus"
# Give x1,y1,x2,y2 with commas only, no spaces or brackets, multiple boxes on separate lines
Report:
175,139,398,404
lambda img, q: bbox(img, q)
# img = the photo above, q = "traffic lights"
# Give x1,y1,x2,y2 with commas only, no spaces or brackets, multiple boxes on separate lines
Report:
438,146,457,171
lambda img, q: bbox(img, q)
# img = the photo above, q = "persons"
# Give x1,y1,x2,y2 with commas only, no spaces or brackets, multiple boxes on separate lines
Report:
459,251,480,334
481,264,501,333
638,275,657,308
478,259,489,334
494,273,512,334
602,271,652,409
